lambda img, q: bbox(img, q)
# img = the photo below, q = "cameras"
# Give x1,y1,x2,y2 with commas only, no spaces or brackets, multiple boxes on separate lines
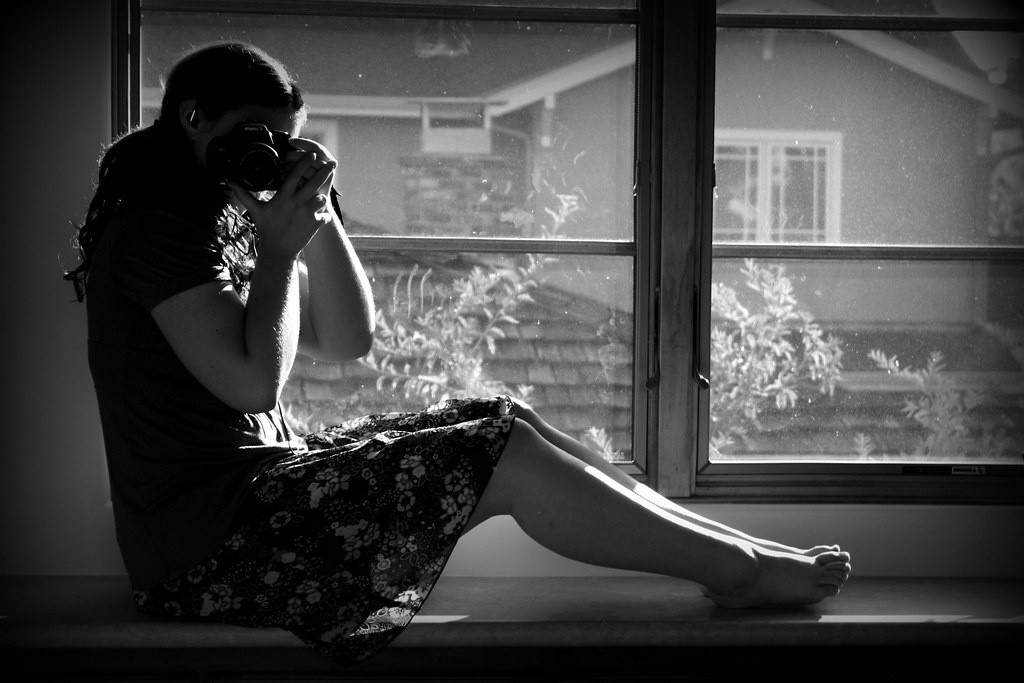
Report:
203,121,303,194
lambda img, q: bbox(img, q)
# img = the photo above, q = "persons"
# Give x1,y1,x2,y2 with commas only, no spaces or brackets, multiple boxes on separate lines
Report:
83,42,852,627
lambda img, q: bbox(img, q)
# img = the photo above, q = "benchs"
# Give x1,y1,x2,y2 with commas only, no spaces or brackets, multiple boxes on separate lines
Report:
0,573,1024,683
